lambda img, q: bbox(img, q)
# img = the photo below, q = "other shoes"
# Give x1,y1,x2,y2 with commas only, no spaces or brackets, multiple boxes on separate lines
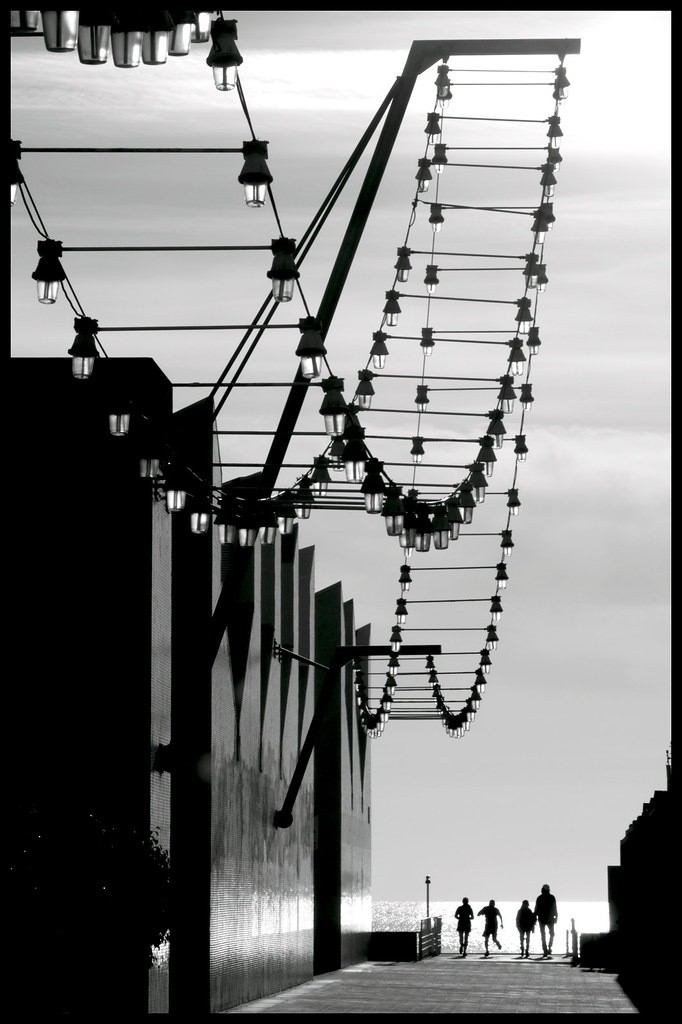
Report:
549,947,551,953
521,952,524,956
485,951,490,956
463,953,467,956
526,952,529,957
497,942,501,949
543,951,547,956
459,946,462,953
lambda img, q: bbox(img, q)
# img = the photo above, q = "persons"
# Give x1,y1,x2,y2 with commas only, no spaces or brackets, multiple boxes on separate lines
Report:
477,899,504,956
455,898,474,956
516,900,535,957
534,884,558,959
